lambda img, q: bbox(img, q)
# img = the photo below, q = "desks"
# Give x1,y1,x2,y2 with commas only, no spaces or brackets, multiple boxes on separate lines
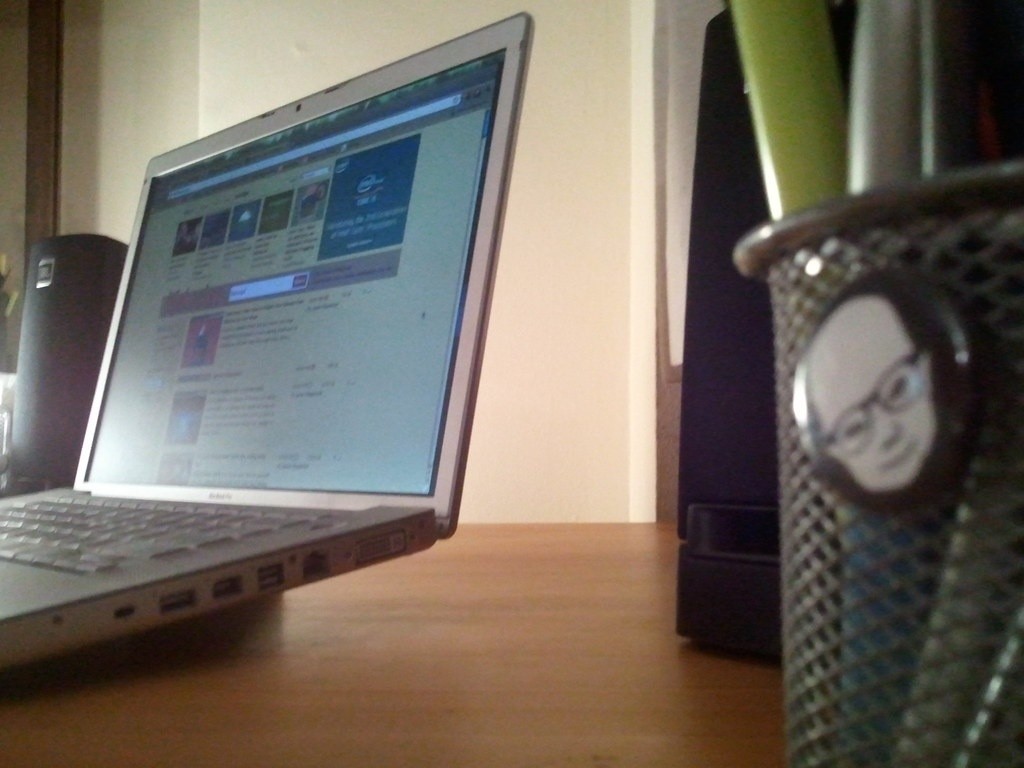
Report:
0,508,1024,768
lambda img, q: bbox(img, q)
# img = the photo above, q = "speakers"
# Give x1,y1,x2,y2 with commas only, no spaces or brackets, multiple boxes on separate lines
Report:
675,0,1024,662
4,233,129,498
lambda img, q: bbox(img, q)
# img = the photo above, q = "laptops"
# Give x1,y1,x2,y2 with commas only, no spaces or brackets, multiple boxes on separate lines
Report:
0,12,534,672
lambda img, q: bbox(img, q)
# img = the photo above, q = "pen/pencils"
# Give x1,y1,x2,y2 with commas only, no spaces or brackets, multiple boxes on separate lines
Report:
728,0,1024,767
